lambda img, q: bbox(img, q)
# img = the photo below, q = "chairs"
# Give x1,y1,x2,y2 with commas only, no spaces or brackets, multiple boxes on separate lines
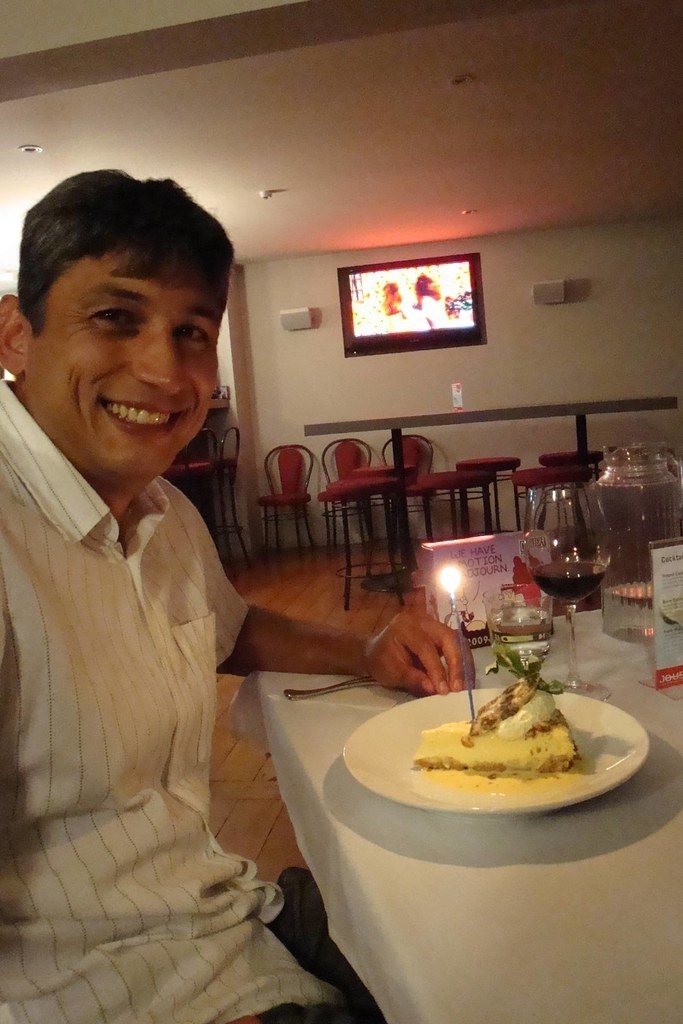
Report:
321,428,435,557
256,444,317,558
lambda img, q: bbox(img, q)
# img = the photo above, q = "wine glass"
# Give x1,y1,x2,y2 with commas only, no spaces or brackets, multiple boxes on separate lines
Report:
526,482,612,701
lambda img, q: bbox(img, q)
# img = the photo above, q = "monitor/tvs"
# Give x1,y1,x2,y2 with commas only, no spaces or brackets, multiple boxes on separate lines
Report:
337,253,487,358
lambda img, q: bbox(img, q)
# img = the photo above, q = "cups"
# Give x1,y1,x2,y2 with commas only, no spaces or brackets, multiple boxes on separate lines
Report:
482,585,552,663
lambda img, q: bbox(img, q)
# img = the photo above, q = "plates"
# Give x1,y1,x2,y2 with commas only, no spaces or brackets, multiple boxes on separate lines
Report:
342,688,650,815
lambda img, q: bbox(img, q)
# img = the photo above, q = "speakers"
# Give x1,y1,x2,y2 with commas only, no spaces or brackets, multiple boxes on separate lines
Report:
532,280,565,305
280,308,312,330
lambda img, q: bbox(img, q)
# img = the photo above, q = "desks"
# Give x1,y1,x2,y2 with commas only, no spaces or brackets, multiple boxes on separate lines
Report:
224,606,683,1024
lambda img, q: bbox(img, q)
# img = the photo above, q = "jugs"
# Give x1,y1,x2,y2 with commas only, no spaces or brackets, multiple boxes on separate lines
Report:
594,442,682,642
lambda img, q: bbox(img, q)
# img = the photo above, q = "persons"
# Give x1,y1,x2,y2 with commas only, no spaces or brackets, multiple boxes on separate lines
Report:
0,169,476,1024
381,273,472,335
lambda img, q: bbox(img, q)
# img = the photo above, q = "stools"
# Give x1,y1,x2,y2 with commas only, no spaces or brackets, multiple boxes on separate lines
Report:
164,424,253,571
319,448,607,608
